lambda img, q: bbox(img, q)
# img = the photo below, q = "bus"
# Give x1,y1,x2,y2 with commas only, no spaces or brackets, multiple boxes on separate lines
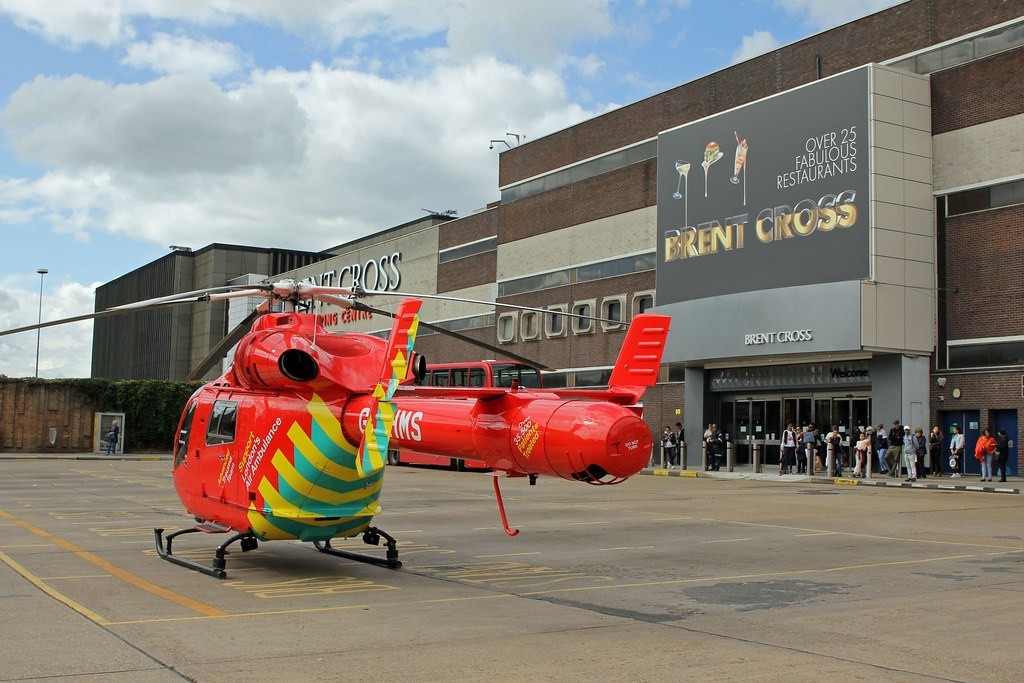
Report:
387,360,545,471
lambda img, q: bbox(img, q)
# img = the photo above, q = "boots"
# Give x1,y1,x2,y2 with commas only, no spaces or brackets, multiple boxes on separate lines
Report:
933,472,941,477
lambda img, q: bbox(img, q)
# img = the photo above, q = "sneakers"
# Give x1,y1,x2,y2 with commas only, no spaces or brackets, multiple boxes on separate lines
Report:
950,473,961,478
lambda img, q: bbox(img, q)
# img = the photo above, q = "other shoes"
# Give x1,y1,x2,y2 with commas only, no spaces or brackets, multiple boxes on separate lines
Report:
106,453,115,455
713,466,720,471
862,474,866,478
705,465,713,470
848,474,861,478
833,470,842,476
784,470,792,474
880,470,888,474
794,471,807,475
818,467,826,471
667,462,675,469
905,478,916,482
998,478,1006,482
886,475,899,479
980,479,993,482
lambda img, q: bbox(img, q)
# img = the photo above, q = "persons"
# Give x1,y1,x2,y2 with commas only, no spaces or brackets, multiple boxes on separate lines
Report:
106,420,119,456
661,419,1009,482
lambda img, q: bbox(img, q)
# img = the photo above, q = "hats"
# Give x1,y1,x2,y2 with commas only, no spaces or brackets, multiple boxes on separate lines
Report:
903,425,911,430
875,423,883,429
893,420,899,424
866,426,874,432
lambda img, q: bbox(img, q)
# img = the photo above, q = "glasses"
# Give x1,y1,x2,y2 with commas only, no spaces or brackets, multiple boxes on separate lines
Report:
796,429,800,430
809,427,813,428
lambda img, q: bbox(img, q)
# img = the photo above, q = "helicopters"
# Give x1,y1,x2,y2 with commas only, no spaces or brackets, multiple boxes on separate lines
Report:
1,277,671,579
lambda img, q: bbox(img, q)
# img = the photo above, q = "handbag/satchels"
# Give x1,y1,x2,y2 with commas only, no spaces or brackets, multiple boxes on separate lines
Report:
949,454,960,470
